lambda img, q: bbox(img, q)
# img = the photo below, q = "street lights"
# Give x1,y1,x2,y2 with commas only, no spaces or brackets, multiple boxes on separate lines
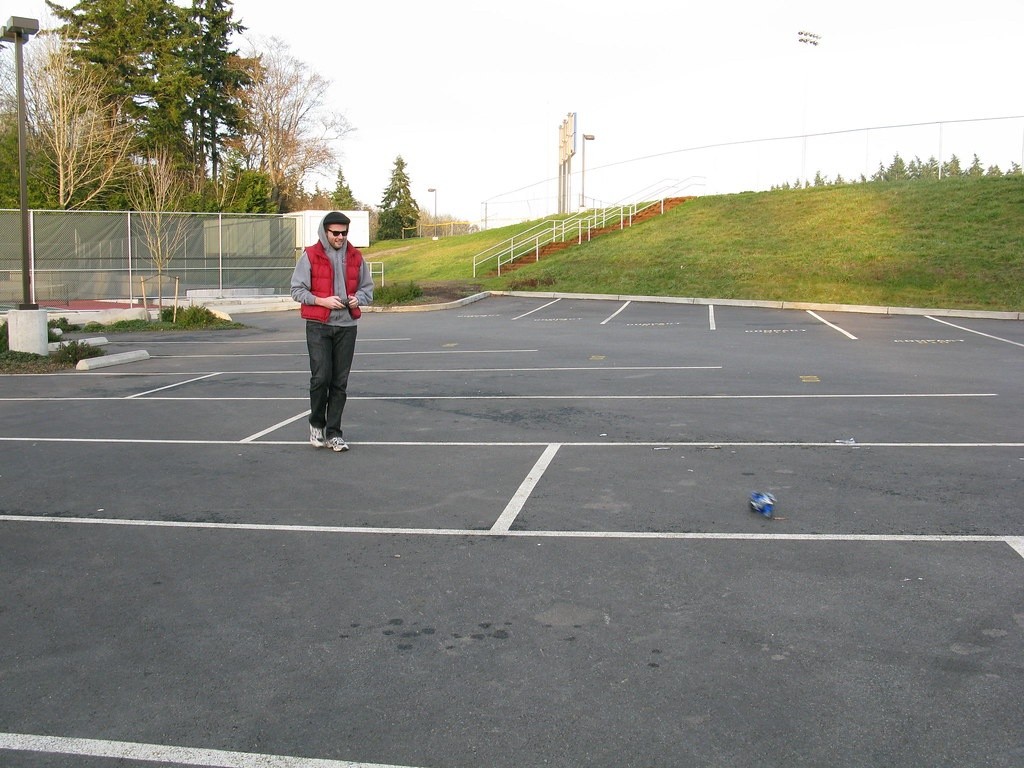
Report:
481,201,488,232
428,188,438,237
582,133,595,208
0,15,42,310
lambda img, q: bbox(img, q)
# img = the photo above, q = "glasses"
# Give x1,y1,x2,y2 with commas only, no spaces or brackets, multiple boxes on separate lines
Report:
327,228,348,236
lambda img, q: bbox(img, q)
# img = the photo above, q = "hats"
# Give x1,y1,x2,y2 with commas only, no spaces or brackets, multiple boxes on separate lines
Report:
324,212,350,225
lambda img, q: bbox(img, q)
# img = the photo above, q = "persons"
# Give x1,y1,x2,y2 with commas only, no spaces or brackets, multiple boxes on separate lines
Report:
290,212,375,454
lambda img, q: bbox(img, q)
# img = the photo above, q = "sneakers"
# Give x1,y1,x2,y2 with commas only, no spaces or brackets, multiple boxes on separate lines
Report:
326,437,349,452
309,422,326,448
